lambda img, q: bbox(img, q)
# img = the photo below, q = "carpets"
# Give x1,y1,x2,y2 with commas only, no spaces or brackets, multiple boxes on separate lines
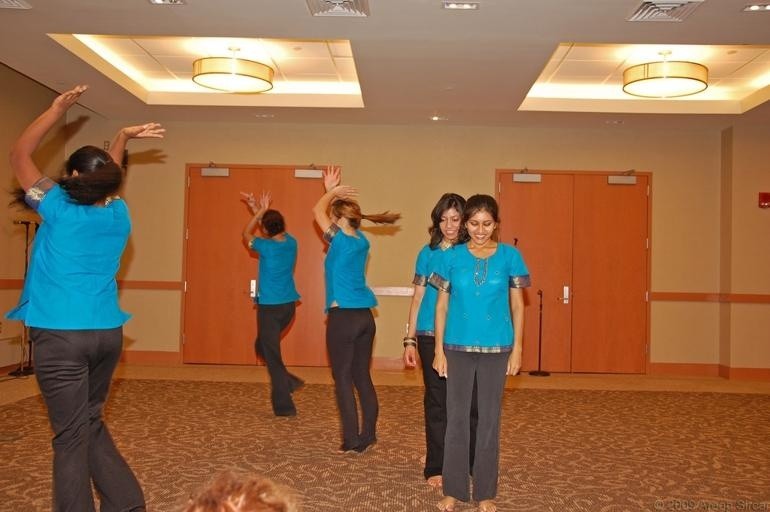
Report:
0,378,770,512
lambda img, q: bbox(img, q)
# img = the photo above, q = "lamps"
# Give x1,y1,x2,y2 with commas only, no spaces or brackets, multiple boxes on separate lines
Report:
623,49,709,98
193,47,274,94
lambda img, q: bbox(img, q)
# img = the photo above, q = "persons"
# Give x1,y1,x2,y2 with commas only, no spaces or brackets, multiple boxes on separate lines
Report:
238,189,306,417
427,195,531,512
12,84,166,512
403,193,478,486
312,163,402,454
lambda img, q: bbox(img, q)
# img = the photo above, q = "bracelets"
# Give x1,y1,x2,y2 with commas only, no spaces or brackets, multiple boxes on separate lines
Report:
401,336,416,348
